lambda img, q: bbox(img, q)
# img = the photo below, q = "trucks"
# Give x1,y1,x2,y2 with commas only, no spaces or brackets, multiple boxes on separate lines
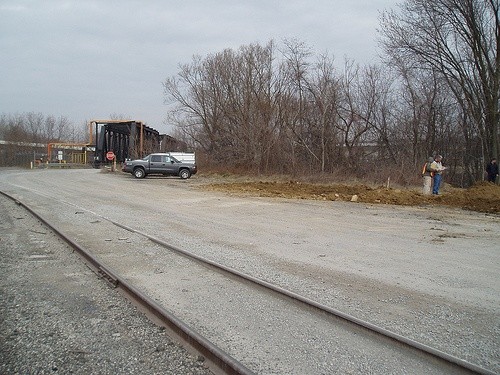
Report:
171,153,196,164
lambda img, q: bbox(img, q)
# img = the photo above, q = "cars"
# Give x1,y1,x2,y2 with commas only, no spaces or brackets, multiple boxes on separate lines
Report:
122,153,197,179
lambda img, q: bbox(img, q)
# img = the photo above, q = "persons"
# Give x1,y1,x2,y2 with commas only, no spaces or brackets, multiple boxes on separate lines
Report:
486,158,499,183
430,155,447,194
422,157,432,194
